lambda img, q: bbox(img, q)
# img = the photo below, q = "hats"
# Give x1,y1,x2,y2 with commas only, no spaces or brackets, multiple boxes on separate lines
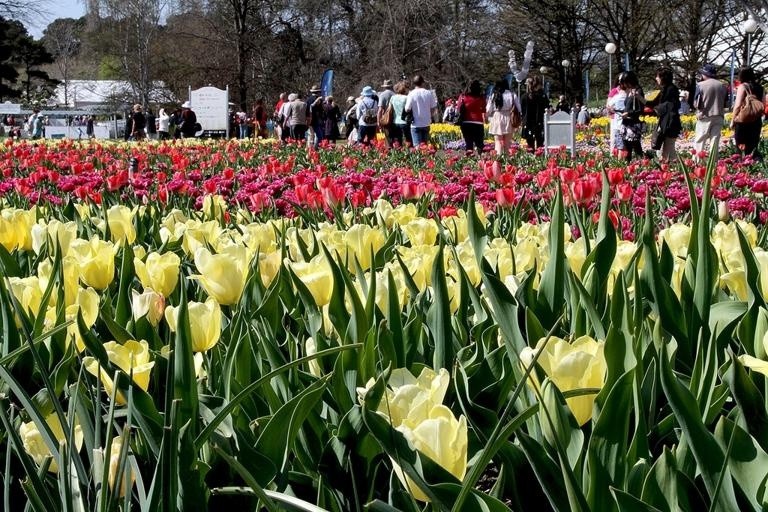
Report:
360,85,377,96
310,85,322,92
698,63,718,79
280,91,286,98
346,95,355,102
180,101,193,108
381,79,393,87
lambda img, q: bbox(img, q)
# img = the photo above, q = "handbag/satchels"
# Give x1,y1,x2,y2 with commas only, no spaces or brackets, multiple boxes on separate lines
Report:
732,82,765,124
453,94,466,126
651,115,665,150
379,105,394,126
401,108,414,123
510,91,521,127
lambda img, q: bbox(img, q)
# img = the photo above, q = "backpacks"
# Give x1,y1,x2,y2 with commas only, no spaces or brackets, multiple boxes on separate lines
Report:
361,99,378,124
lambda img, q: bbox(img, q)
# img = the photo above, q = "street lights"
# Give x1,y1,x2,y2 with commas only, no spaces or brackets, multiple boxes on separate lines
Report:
561,58,570,100
740,14,757,66
604,42,617,92
538,64,548,88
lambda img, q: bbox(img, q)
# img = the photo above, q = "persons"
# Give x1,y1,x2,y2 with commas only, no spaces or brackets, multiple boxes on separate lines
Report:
606,72,629,161
632,68,682,164
726,67,768,155
694,64,728,164
608,70,645,161
